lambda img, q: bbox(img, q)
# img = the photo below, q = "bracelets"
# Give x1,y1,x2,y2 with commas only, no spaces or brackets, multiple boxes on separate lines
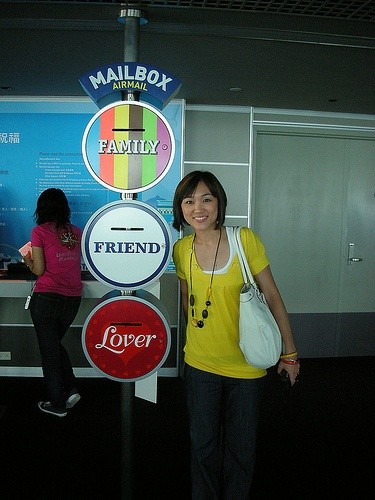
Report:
280,352,297,360
281,358,299,364
25,259,31,264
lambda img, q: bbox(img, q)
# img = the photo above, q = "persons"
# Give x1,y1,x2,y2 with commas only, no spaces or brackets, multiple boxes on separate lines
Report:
167,171,301,500
23,187,90,417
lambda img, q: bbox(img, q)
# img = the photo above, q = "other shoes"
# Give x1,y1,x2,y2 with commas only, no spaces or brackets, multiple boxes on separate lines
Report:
65,391,80,409
38,401,67,417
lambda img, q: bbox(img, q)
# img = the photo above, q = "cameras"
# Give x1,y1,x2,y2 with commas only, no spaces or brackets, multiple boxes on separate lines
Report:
25,295,31,310
280,369,290,384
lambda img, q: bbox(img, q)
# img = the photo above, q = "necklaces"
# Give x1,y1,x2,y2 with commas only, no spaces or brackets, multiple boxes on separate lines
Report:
188,224,226,328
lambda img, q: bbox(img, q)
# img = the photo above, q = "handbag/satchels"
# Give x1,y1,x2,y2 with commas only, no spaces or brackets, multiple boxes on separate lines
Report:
232,226,282,369
